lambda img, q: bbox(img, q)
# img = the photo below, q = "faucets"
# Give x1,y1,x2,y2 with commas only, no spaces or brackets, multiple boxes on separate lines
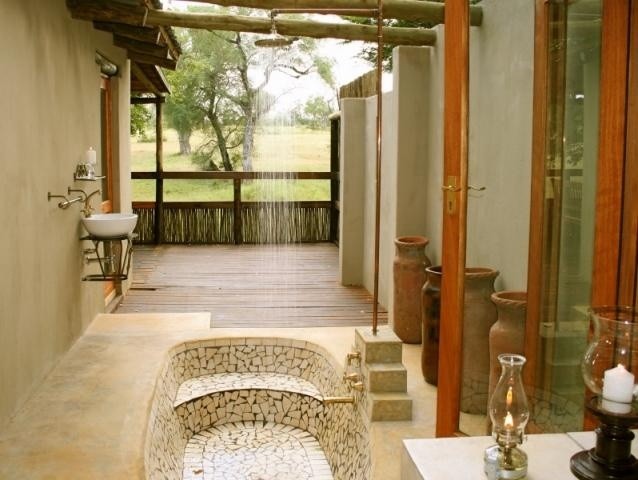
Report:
84,189,101,216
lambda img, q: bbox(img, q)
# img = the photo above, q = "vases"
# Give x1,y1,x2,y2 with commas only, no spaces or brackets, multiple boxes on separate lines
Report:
393,236,526,433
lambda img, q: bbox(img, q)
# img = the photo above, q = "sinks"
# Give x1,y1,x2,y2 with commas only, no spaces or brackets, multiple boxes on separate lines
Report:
81,213,139,238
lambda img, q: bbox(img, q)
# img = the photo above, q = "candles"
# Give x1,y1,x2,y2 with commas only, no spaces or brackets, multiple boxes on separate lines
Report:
600,363,635,413
